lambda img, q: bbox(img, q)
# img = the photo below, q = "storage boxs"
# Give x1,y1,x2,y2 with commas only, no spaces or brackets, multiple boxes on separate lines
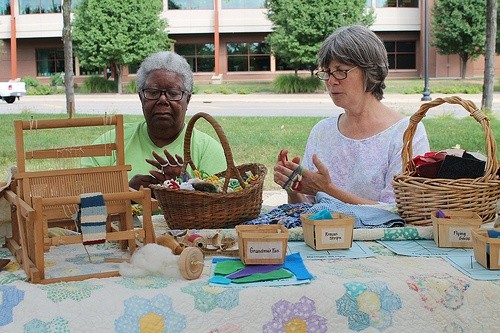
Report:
431,211,482,248
471,227,500,271
299,213,354,251
236,225,288,266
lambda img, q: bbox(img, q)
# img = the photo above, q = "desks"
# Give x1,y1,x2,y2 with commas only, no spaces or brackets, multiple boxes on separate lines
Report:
0,197,500,333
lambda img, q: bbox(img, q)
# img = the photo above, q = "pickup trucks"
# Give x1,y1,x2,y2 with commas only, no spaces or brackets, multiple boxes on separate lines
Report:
0,82,26,104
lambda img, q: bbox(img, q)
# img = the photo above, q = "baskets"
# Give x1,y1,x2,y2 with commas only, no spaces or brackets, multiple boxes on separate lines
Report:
148,112,267,230
391,96,500,227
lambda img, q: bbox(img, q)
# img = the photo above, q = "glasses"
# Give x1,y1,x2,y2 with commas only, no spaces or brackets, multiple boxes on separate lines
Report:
315,65,359,80
142,88,185,101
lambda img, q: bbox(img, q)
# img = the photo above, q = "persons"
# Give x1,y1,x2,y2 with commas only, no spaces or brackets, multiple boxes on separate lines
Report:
274,26,430,206
77,51,227,205
